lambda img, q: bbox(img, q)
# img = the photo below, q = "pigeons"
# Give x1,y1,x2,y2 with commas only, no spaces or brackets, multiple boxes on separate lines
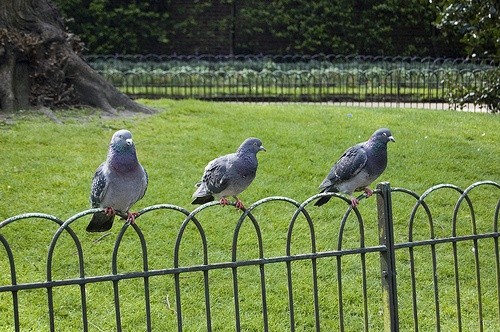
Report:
85,129,149,233
314,128,396,208
190,137,267,209
